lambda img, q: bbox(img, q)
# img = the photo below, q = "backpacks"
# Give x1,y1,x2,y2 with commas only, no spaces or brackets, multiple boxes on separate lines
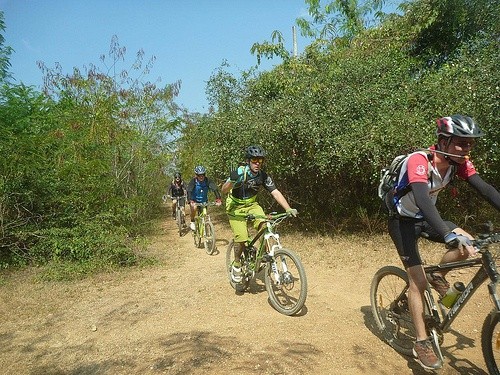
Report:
378,151,458,216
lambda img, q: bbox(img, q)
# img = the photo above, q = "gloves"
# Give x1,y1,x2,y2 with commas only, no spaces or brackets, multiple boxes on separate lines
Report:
228,163,246,182
286,208,299,219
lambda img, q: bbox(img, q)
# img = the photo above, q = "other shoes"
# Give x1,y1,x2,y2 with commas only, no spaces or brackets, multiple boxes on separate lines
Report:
232,263,244,283
190,220,196,231
172,213,176,220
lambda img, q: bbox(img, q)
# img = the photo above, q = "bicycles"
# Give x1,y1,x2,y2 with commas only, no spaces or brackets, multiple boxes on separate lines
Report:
370,232,500,375
188,201,219,255
225,211,307,316
167,196,187,237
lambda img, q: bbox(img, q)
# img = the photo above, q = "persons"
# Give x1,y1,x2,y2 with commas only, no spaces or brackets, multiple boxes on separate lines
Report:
167,173,188,228
388,115,500,369
223,146,298,281
188,166,222,243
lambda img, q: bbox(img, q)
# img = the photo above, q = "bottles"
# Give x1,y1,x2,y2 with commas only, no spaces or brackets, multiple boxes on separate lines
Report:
441,281,465,309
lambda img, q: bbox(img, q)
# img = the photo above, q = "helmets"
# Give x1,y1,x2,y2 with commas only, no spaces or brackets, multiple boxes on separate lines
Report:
174,172,181,177
436,115,486,139
244,145,267,158
194,166,206,175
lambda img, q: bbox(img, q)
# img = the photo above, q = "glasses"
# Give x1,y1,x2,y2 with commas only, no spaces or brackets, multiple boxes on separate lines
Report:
249,157,264,164
175,177,181,179
448,141,476,151
197,174,204,177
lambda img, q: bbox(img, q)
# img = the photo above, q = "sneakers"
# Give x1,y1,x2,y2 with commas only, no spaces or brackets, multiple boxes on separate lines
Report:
412,339,442,371
427,270,451,298
